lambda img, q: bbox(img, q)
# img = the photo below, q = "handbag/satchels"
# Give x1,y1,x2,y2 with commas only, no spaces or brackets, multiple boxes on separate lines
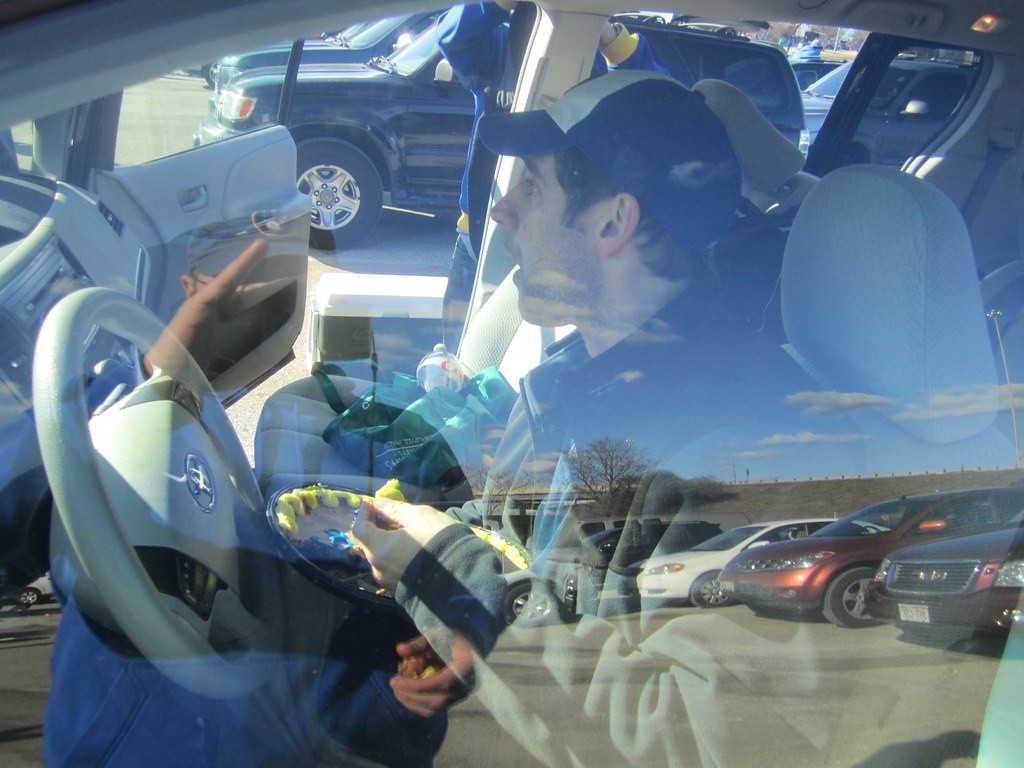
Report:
311,352,519,494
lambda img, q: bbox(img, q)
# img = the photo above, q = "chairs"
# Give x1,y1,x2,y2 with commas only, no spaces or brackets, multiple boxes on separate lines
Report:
777,167,1023,768
253,77,806,500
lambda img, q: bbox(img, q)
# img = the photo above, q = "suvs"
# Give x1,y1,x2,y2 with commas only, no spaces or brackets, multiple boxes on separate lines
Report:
193,10,976,253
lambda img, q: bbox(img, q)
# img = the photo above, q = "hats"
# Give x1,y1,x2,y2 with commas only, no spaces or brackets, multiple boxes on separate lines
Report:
185,195,336,270
477,70,741,254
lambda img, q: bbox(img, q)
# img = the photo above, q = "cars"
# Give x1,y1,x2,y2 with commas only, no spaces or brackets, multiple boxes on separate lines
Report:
1,567,53,606
717,485,1024,629
867,510,1024,645
493,518,725,626
615,518,891,608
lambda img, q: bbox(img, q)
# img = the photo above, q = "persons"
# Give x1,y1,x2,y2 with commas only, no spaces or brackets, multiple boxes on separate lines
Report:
346,69,894,768
0,192,514,768
433,0,676,319
791,39,823,61
